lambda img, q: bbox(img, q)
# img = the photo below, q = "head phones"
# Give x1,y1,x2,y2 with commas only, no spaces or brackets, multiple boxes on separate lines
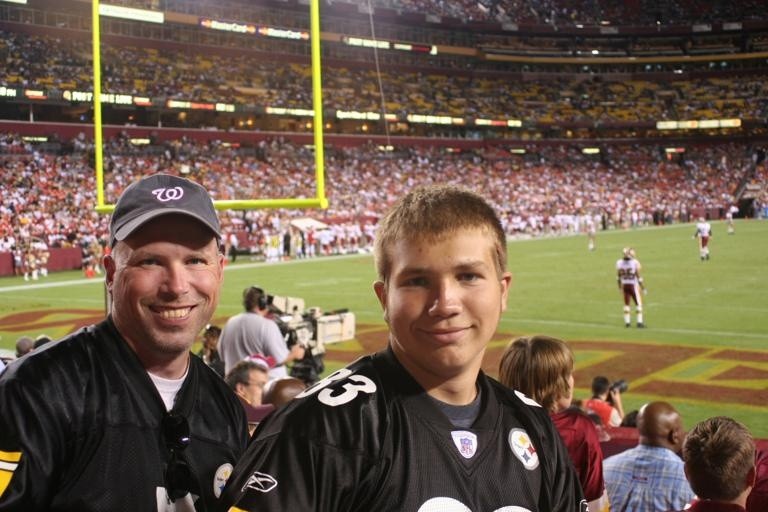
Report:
242,286,269,311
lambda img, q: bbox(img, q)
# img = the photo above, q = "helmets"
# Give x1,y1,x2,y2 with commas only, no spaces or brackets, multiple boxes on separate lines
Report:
620,247,637,260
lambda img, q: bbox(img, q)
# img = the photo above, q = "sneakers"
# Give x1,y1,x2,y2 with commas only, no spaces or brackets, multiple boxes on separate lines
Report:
624,323,644,328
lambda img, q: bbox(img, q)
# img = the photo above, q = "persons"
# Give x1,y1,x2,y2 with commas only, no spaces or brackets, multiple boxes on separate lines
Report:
601,399,695,512
222,183,589,512
681,414,758,512
498,333,608,512
0,174,250,512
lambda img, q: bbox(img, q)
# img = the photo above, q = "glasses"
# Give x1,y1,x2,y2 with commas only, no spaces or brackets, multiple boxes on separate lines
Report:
157,409,193,503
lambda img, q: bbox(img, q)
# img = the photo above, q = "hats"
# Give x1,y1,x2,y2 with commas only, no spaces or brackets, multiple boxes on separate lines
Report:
109,172,222,242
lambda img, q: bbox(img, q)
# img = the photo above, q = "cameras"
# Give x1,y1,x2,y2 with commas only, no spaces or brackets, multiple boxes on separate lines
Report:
605,378,628,399
